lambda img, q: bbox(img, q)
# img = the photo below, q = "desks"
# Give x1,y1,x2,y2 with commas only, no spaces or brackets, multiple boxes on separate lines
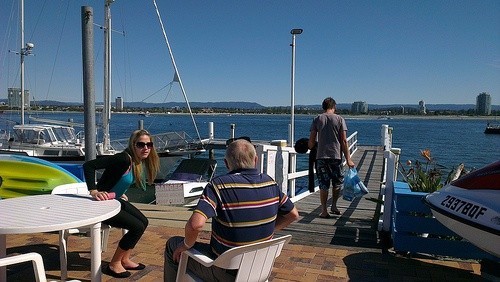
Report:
0,193,122,282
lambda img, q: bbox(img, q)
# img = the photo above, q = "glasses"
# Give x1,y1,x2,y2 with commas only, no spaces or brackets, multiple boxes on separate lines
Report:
136,142,153,149
226,136,252,148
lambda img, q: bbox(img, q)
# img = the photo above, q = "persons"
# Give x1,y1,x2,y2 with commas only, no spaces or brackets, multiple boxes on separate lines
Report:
82,128,161,278
164,137,300,282
307,97,355,219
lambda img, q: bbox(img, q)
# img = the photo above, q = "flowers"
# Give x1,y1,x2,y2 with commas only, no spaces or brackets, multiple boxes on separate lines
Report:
406,149,438,192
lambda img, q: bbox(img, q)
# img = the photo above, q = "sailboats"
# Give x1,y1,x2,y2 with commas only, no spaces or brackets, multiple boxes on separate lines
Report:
0,0,206,183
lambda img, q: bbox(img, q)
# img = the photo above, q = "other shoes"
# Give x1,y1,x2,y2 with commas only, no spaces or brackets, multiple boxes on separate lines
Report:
102,263,131,278
125,263,146,270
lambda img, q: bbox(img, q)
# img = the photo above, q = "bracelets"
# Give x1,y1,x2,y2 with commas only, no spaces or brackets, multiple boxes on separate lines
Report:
183,238,194,249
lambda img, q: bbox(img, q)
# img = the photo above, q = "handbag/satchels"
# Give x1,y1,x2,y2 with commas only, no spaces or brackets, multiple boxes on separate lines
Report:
343,166,369,201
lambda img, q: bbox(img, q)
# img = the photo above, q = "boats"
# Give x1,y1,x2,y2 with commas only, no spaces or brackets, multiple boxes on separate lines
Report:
425,160,499,259
0,154,84,200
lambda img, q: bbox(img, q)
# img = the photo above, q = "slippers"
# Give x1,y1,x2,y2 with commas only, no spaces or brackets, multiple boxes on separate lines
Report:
329,209,342,215
319,213,330,218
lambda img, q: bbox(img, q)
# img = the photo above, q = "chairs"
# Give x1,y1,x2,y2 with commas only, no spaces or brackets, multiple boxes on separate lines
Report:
0,251,82,282
173,234,292,282
51,181,129,254
